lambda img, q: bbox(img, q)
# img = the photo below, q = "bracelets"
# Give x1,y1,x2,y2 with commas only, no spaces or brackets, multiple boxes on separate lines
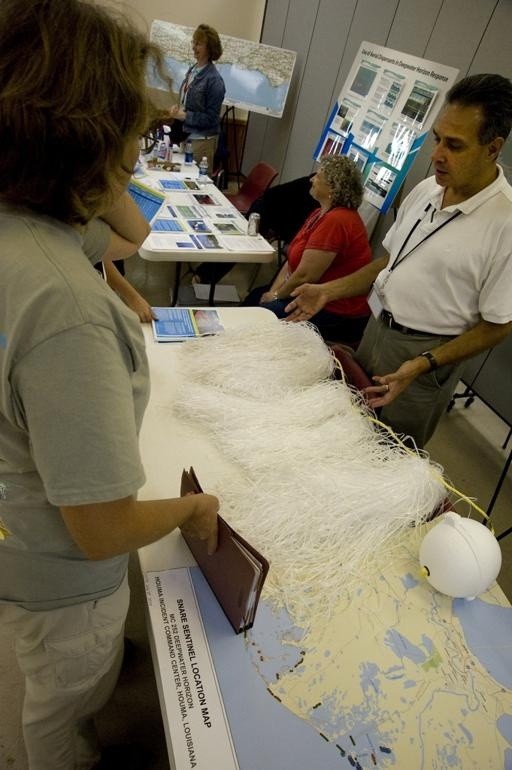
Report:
417,350,438,370
271,290,278,301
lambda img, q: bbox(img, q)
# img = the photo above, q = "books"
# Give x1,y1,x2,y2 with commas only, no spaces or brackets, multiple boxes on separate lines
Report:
176,464,270,638
149,306,225,345
157,180,203,193
126,178,168,226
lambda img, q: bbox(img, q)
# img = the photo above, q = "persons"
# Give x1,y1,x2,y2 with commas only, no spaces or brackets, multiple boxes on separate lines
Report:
279,71,511,458
0,1,221,770
169,24,226,179
241,152,373,351
93,259,160,323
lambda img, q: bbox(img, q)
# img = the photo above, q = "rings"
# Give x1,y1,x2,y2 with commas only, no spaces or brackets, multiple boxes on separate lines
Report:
385,384,389,392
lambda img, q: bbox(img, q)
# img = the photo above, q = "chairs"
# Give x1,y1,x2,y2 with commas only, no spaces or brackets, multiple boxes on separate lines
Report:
222,160,279,215
328,343,382,419
209,163,229,192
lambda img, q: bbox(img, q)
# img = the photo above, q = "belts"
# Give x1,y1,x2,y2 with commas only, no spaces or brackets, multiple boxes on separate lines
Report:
380,312,457,338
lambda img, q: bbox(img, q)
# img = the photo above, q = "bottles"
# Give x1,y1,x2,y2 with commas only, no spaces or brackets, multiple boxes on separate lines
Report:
197,157,208,185
184,140,193,166
152,144,158,156
166,143,173,161
160,142,165,159
156,128,160,139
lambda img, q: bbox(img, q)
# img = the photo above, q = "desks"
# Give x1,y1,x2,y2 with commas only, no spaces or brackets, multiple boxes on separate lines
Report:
130,153,276,307
137,306,512,770
